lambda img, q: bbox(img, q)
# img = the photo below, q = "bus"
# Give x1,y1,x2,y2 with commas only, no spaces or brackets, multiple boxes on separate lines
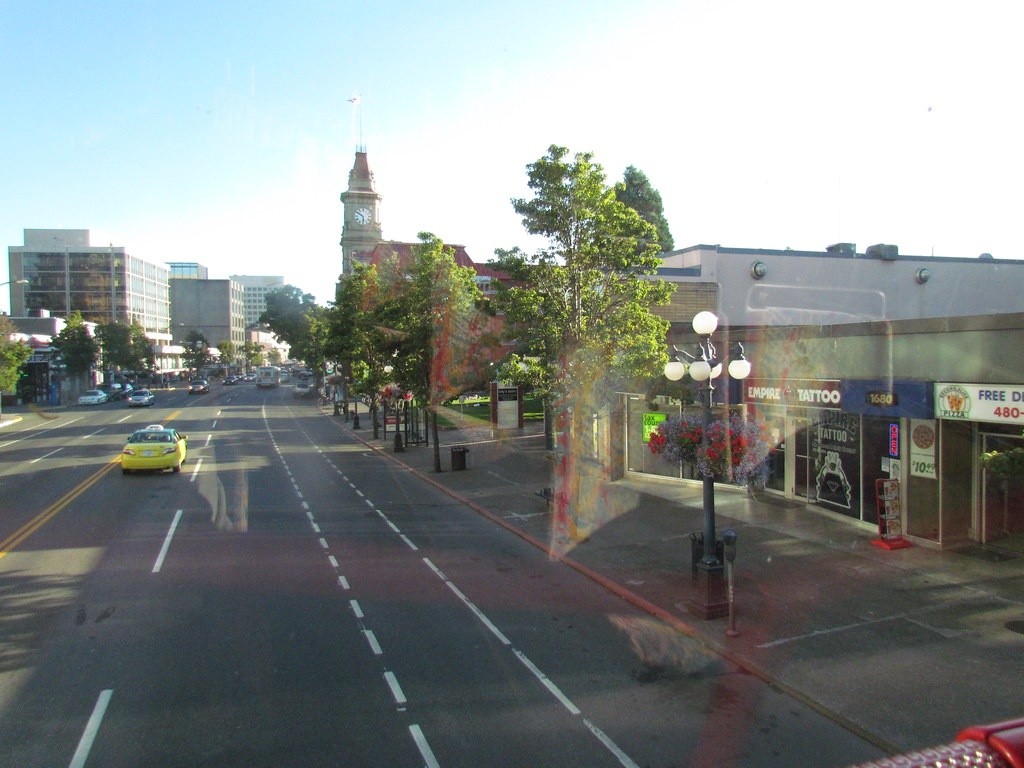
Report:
255,365,282,389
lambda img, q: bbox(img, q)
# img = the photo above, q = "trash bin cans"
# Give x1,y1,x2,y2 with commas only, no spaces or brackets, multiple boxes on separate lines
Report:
450,445,468,470
689,532,725,580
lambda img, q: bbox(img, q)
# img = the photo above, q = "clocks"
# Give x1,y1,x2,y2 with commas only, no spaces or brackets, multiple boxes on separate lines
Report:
353,207,373,226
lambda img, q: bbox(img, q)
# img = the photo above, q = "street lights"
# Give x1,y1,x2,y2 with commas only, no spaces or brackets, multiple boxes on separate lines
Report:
663,310,752,621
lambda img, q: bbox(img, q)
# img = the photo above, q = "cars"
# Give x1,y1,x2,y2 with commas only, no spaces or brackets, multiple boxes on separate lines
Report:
188,379,210,395
94,383,134,402
279,359,314,396
522,387,561,414
121,424,189,473
127,389,155,406
77,390,108,406
323,360,336,388
233,372,257,382
222,375,239,385
